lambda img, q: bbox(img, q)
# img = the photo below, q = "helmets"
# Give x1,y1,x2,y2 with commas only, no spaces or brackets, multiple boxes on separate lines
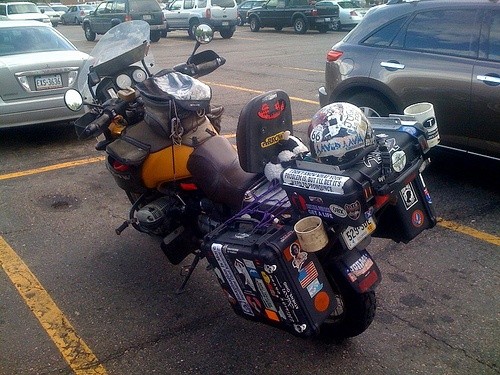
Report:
307,102,374,161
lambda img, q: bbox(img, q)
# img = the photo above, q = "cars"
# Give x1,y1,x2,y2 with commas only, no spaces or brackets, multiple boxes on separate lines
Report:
237,0,369,34
0,1,54,29
37,0,114,25
0,19,95,133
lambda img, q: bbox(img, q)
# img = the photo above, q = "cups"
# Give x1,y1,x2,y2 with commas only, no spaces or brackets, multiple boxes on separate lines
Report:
403,103,441,148
293,216,327,252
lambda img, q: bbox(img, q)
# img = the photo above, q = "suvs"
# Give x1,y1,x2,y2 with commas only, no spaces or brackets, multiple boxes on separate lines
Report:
80,0,170,43
316,0,499,177
162,0,239,41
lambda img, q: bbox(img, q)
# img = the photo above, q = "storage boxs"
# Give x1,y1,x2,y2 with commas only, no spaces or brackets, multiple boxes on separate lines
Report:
368,171,444,246
204,215,341,336
279,111,434,225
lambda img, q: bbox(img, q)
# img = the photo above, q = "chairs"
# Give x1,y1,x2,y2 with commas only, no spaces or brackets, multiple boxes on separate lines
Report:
184,86,295,198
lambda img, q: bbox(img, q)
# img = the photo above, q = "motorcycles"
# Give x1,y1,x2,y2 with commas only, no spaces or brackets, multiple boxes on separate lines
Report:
63,19,442,342
